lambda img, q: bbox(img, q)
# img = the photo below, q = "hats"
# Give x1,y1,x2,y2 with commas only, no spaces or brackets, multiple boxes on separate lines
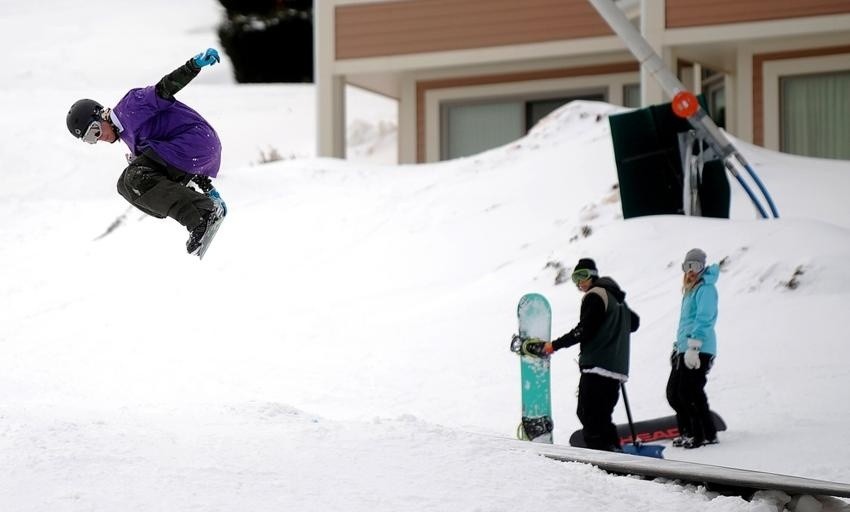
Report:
574,259,598,279
685,248,706,265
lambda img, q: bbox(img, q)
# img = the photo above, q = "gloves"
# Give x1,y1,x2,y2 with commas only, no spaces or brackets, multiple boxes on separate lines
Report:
193,48,220,68
684,338,702,369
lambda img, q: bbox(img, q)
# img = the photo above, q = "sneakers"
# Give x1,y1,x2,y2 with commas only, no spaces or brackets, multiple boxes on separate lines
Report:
187,204,223,256
673,431,717,448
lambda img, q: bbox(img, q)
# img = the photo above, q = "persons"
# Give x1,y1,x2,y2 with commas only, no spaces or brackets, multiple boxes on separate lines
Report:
663,246,721,452
516,255,641,451
64,46,230,260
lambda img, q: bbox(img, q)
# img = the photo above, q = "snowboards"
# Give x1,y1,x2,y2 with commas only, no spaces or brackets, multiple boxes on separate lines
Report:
571,412,727,451
198,190,227,259
517,295,554,444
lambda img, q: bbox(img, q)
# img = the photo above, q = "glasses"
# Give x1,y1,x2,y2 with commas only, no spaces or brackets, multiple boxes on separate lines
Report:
682,262,702,273
82,121,102,144
571,269,593,281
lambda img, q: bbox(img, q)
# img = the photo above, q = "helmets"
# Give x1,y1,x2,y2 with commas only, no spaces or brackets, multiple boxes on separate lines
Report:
66,99,105,139
